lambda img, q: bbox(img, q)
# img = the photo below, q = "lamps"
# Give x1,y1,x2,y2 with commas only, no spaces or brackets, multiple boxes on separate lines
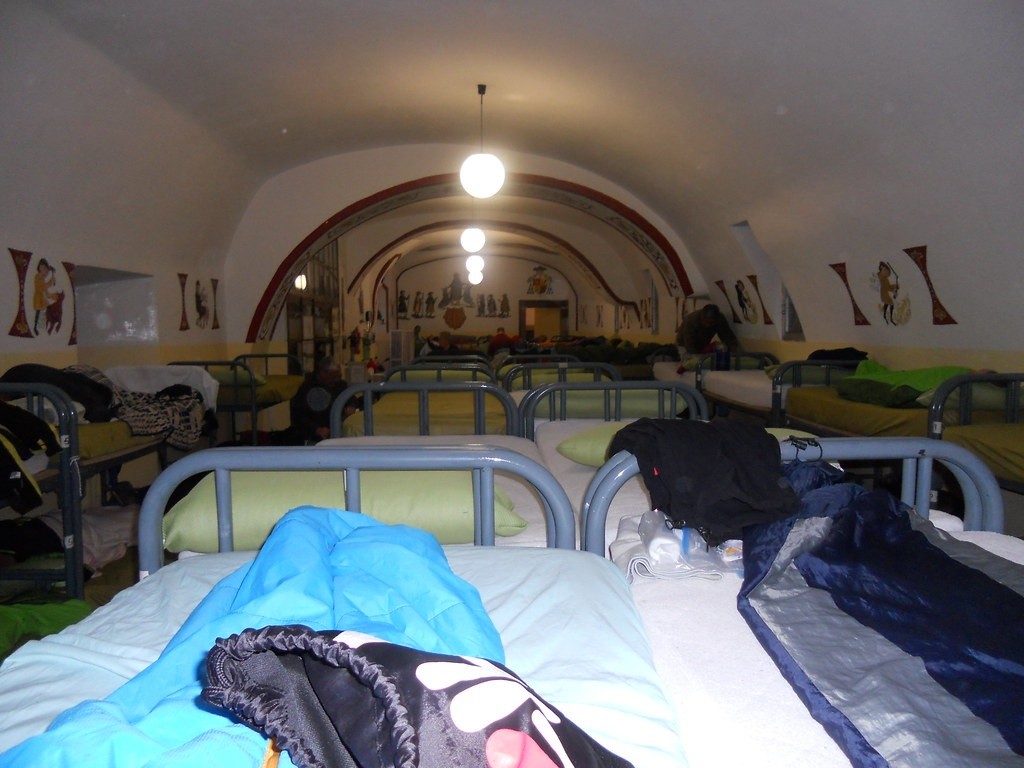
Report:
468,272,483,284
460,84,505,198
460,228,486,253
466,255,484,271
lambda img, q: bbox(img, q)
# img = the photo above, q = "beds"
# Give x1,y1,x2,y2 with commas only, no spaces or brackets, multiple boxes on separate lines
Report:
1,333,1024,768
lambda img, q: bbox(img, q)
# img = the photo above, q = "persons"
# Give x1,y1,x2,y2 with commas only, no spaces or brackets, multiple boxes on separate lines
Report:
675,304,740,365
427,331,460,356
290,357,359,447
488,327,516,357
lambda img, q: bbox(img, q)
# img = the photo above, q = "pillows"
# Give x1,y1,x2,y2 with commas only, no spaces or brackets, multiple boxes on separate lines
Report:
162,470,529,552
765,363,855,385
557,420,820,466
534,388,687,418
388,370,492,383
512,372,612,390
495,363,584,379
205,368,266,385
680,353,763,369
915,379,1024,411
